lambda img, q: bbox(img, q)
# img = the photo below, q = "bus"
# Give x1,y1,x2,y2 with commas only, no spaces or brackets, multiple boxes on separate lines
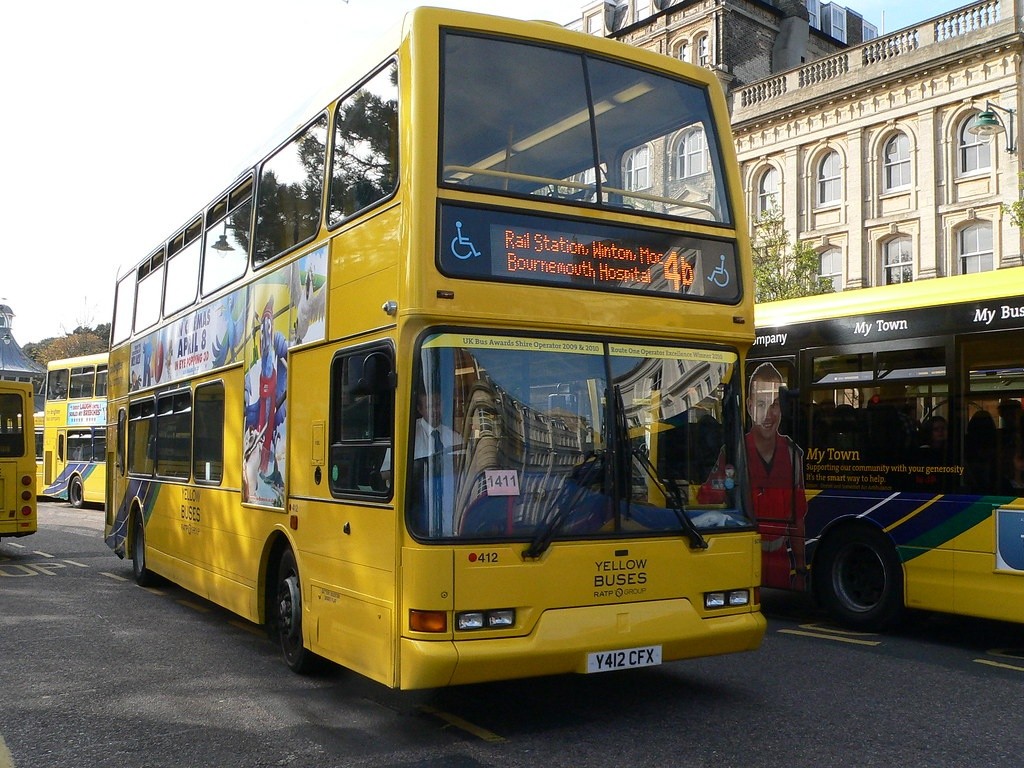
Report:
0,380,38,542
6,414,47,497
105,5,767,690
42,351,222,510
641,265,1024,633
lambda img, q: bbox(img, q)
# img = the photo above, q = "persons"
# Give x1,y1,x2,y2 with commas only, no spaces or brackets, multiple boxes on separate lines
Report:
696,360,808,589
699,397,1024,495
381,387,471,487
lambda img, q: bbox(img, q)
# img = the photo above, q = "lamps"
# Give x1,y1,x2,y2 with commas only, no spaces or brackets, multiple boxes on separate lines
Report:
211,219,235,257
967,99,1013,155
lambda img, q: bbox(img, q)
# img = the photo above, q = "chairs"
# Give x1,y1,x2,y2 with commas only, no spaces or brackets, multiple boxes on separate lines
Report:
72,383,107,397
967,410,996,467
